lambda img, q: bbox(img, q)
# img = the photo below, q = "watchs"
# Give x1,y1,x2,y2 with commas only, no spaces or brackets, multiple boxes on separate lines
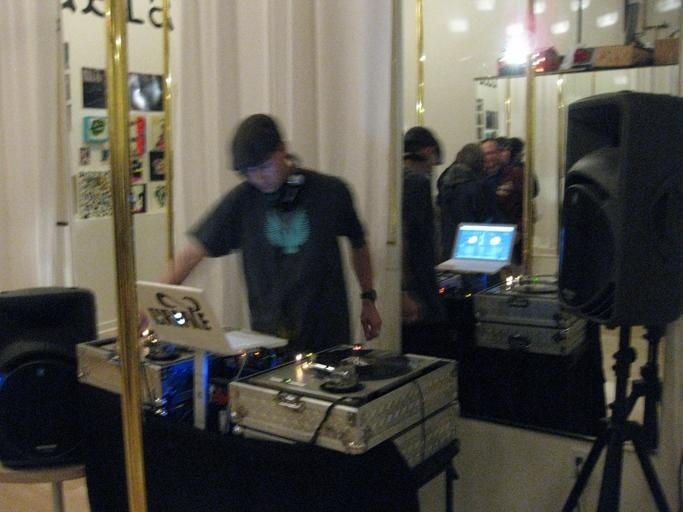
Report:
360,289,377,301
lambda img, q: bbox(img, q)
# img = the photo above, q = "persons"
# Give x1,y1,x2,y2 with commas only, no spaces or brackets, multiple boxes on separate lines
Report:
111,113,382,364
400,125,538,356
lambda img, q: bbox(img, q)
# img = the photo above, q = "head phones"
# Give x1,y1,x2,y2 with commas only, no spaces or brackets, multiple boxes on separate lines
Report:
285,151,306,187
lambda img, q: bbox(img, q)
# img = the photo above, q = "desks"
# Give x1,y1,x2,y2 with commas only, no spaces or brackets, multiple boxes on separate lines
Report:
80,387,461,512
420,336,603,428
1,465,86,511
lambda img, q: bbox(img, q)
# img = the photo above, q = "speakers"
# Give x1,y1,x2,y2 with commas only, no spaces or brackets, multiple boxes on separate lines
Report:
557,90,683,326
1,286,97,470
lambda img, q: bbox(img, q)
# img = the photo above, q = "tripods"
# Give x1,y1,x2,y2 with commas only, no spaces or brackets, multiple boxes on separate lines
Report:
561,327,670,512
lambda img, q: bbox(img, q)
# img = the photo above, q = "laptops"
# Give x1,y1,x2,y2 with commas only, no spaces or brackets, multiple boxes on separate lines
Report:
433,221,518,275
136,281,290,357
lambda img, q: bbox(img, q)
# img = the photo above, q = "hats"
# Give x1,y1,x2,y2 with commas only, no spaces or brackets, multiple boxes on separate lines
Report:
232,115,278,172
405,126,441,165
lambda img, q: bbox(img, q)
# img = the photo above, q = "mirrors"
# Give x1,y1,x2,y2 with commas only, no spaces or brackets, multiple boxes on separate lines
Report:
401,0,682,453
1,0,683,512
